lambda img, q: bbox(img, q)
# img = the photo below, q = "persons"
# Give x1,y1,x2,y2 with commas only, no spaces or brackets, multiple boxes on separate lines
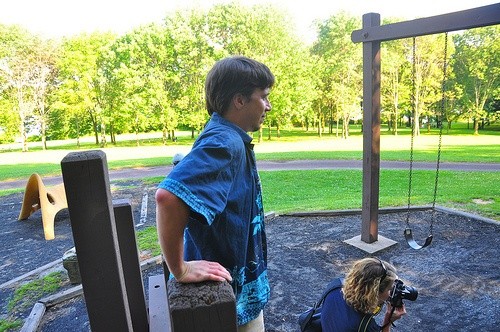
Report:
153,55,272,332
320,256,406,332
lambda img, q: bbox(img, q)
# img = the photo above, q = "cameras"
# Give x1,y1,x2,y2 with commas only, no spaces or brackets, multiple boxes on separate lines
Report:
386,279,419,308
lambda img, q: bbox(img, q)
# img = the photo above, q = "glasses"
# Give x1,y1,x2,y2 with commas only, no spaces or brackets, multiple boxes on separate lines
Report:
371,256,388,284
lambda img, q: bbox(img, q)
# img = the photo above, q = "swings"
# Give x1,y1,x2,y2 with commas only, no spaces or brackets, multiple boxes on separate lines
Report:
404,31,448,250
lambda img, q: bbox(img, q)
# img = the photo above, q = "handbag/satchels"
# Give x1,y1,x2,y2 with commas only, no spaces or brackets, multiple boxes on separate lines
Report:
298,306,322,332
